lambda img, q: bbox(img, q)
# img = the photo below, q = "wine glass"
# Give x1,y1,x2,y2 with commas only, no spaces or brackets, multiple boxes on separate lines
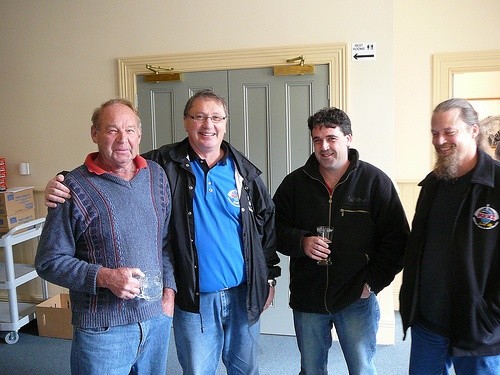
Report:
316,226,334,266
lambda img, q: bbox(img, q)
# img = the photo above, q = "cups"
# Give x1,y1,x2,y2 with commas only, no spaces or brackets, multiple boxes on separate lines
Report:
133,270,163,302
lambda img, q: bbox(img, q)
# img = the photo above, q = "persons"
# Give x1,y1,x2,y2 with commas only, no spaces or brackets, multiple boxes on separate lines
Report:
272,107,411,375
398,98,500,375
35,89,282,375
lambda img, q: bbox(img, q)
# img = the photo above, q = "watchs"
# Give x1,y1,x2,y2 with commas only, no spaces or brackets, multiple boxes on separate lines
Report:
267,278,276,287
365,282,374,294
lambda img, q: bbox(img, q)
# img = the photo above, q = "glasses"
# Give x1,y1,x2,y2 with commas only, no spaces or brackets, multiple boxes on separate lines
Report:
187,113,227,123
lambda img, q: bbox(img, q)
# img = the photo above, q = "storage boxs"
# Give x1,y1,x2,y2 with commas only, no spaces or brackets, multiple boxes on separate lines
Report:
0,187,35,238
36,293,73,340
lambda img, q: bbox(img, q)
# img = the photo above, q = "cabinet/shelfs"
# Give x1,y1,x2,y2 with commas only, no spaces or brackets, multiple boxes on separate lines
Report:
0,217,48,344
